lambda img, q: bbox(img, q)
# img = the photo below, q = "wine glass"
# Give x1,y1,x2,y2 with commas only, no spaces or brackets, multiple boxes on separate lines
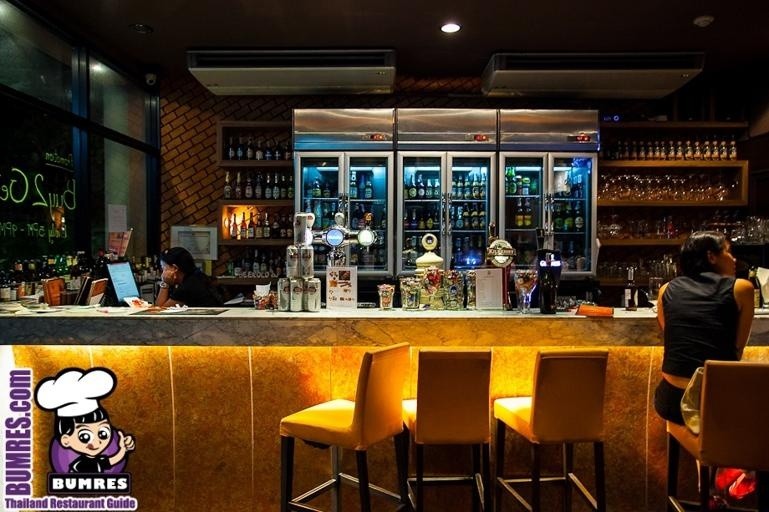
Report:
597,174,740,202
681,213,769,244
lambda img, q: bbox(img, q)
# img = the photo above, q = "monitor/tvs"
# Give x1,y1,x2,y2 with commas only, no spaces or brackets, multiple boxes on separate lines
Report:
106,260,141,303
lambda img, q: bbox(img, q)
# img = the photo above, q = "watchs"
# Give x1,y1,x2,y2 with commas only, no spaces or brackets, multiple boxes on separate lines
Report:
159,281,168,289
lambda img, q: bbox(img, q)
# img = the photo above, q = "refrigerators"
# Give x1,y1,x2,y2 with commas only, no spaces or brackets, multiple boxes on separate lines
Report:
395,107,497,276
290,106,395,281
497,108,602,282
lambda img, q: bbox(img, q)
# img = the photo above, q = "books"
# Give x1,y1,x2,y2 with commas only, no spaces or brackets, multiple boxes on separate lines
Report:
41,277,109,306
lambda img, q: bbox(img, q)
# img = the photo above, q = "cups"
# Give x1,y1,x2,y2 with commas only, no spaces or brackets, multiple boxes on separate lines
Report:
648,276,664,300
558,292,592,309
203,261,212,277
598,254,676,279
596,214,666,239
376,269,539,314
254,291,278,310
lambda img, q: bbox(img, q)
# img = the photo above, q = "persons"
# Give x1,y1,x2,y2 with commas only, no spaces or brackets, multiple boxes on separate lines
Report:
156,247,224,308
655,230,755,427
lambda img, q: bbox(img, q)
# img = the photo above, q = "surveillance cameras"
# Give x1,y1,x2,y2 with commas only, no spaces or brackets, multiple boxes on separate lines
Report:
146,74,157,86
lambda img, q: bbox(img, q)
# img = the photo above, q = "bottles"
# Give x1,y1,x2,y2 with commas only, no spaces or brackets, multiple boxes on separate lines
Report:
506,165,584,270
403,173,487,266
748,266,762,308
305,170,387,265
0,249,160,302
599,140,737,160
224,136,294,278
666,215,674,239
540,253,558,315
624,269,639,311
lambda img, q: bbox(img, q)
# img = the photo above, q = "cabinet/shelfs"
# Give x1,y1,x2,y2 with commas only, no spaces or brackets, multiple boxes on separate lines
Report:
597,159,749,291
215,121,294,286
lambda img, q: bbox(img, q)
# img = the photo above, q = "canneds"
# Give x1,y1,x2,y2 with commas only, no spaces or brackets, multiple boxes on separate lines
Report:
304,278,321,312
286,245,301,280
301,246,314,280
277,278,290,311
290,280,305,312
293,213,307,245
303,279,310,311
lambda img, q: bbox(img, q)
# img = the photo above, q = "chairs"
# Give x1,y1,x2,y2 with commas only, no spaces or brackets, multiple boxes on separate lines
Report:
494,349,608,512
666,359,769,512
402,349,493,512
278,342,411,512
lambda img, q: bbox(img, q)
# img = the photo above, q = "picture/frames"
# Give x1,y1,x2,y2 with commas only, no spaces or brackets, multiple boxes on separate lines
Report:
170,225,217,260
